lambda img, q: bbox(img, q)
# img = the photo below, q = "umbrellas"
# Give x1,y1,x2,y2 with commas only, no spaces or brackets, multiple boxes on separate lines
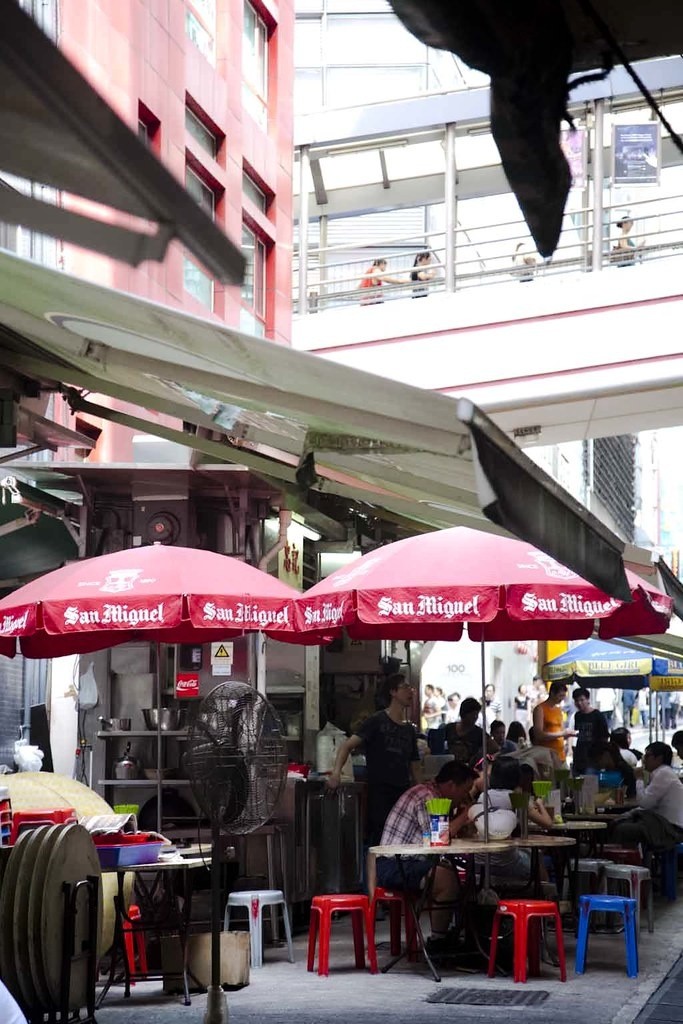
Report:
0,541,344,832
294,525,675,885
542,639,683,744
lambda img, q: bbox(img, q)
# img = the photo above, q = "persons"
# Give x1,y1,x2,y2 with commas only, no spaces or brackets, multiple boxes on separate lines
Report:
571,688,609,773
513,684,531,742
434,688,444,697
421,684,442,736
375,697,683,973
596,687,683,732
614,216,644,268
529,675,548,710
477,683,502,736
411,252,441,299
322,673,421,846
534,682,578,767
445,691,460,723
510,243,537,281
355,259,411,307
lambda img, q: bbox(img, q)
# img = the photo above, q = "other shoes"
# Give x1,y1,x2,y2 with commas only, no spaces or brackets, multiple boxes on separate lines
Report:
424,935,458,968
462,947,490,967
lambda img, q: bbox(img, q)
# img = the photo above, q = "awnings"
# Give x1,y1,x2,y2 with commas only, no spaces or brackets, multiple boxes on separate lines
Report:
0,246,630,601
302,495,682,623
1,0,247,285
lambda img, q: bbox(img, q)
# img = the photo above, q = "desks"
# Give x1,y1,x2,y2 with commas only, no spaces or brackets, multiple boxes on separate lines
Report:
95,857,211,1009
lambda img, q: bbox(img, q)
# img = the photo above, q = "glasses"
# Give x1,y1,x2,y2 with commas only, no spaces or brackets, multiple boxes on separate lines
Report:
645,754,653,760
398,684,416,691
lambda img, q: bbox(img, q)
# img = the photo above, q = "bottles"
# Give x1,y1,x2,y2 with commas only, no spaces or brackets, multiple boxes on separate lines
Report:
597,769,609,794
516,737,527,750
423,833,430,847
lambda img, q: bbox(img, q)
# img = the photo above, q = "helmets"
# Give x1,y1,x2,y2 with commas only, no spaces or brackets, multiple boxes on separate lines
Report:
468,803,518,840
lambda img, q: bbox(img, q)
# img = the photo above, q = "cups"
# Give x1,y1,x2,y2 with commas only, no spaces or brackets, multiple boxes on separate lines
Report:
614,784,624,804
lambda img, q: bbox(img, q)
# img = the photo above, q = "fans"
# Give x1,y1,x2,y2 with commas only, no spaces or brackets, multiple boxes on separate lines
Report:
183,681,288,1024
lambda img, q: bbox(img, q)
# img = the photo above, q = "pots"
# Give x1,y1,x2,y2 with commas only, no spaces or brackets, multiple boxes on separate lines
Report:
98,716,132,731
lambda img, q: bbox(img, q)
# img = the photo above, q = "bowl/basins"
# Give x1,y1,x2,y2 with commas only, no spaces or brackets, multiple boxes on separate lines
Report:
141,708,181,731
144,769,169,779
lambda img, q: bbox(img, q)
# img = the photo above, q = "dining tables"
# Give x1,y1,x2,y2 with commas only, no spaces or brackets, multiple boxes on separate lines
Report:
0,825,102,1024
368,799,642,974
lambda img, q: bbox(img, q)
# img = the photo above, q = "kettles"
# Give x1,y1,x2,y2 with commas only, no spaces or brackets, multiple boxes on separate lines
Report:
111,741,139,780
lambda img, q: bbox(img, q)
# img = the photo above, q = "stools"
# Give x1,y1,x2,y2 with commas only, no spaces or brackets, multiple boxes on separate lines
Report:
308,895,374,973
488,900,566,982
121,905,148,986
0,787,79,843
571,844,683,979
372,887,417,953
226,889,294,969
